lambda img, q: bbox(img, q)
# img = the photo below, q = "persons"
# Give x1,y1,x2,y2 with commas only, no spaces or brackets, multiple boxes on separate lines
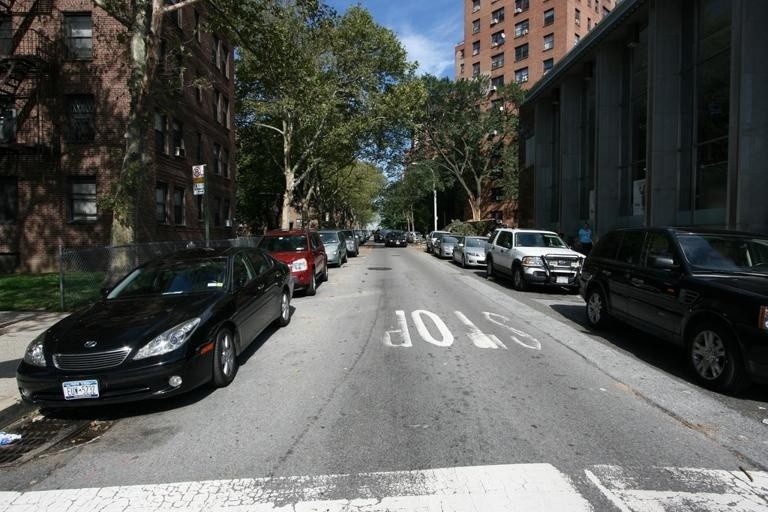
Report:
576,221,594,256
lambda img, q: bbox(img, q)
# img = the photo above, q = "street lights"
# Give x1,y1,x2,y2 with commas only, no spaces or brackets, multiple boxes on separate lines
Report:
412,162,439,231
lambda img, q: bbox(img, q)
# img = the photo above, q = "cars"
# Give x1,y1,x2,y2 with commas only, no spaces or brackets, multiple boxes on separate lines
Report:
17,248,294,409
317,229,370,265
427,231,489,267
375,228,421,248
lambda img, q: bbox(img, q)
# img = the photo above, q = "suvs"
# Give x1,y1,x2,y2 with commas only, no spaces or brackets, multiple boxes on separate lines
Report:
257,230,329,296
581,226,767,391
484,227,586,289
